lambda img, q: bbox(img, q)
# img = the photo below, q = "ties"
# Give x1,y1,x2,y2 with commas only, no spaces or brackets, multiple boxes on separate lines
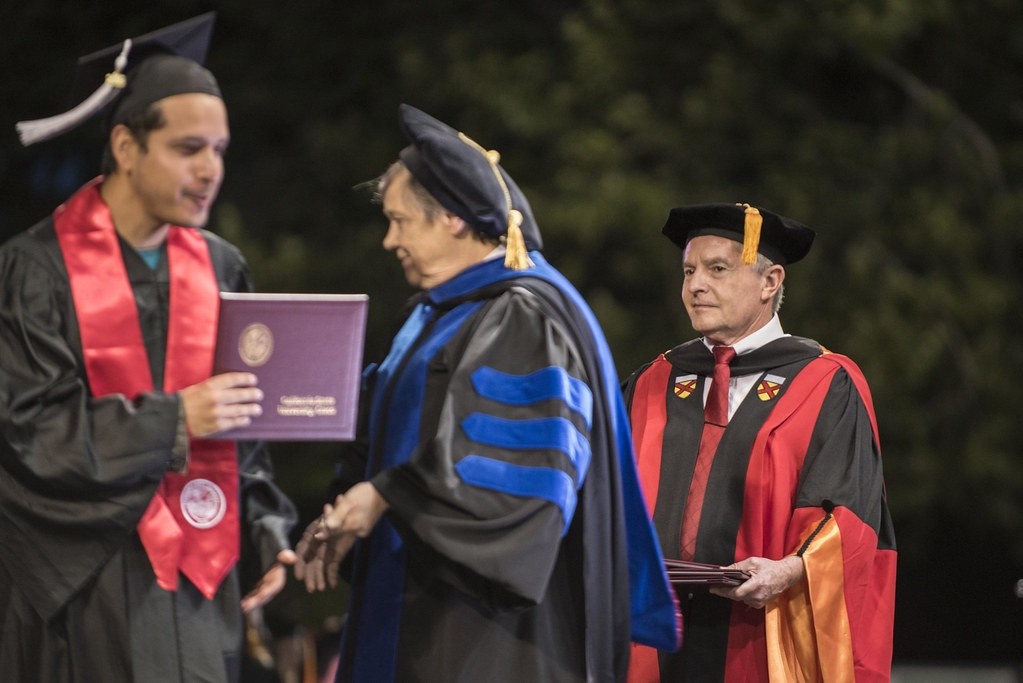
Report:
679,346,737,562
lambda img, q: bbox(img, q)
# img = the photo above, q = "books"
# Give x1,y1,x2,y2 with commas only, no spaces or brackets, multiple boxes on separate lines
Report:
199,289,368,440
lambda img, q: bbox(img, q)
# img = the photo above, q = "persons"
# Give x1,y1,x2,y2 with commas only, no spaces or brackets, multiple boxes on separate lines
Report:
1,12,294,683
619,203,898,683
294,105,682,683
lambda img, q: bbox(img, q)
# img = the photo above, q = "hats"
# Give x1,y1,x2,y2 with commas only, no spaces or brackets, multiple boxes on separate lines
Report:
397,102,544,272
15,11,224,147
662,202,818,264
